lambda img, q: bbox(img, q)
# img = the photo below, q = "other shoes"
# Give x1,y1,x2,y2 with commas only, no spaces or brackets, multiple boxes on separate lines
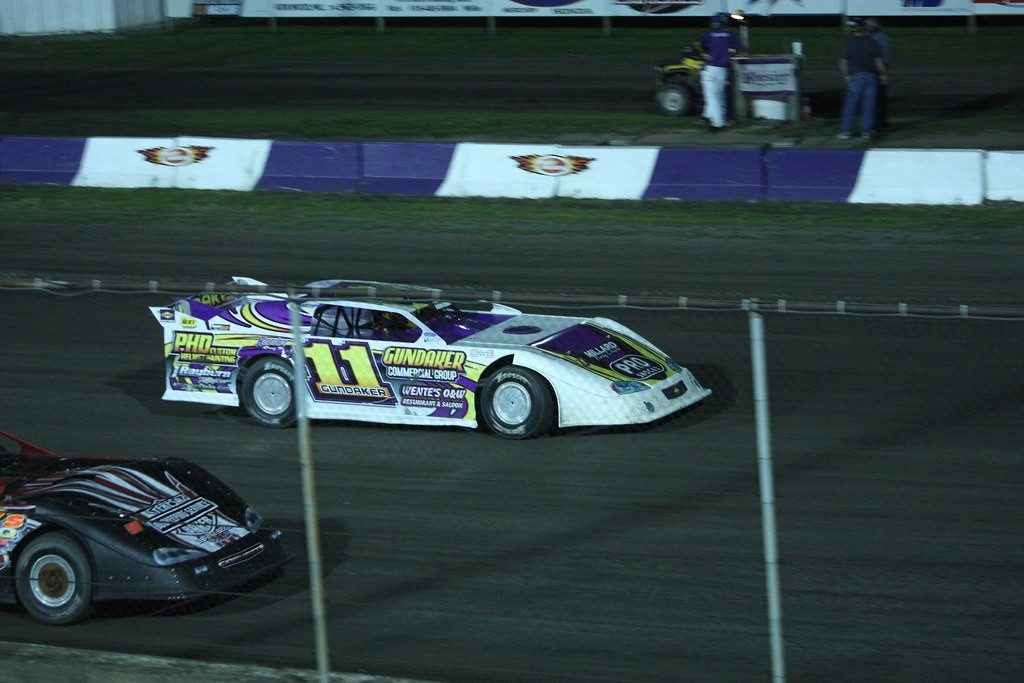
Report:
709,125,726,131
859,132,874,140
697,116,709,120
835,132,851,139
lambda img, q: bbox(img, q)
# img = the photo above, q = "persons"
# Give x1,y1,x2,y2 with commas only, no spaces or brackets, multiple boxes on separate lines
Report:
694,12,750,132
835,17,892,140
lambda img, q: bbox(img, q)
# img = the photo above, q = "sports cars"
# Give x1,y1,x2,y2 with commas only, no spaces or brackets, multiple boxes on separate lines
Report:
0,429,291,627
147,276,713,442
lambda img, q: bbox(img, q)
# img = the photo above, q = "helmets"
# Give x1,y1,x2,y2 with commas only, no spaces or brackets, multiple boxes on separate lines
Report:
710,15,728,29
847,16,863,32
